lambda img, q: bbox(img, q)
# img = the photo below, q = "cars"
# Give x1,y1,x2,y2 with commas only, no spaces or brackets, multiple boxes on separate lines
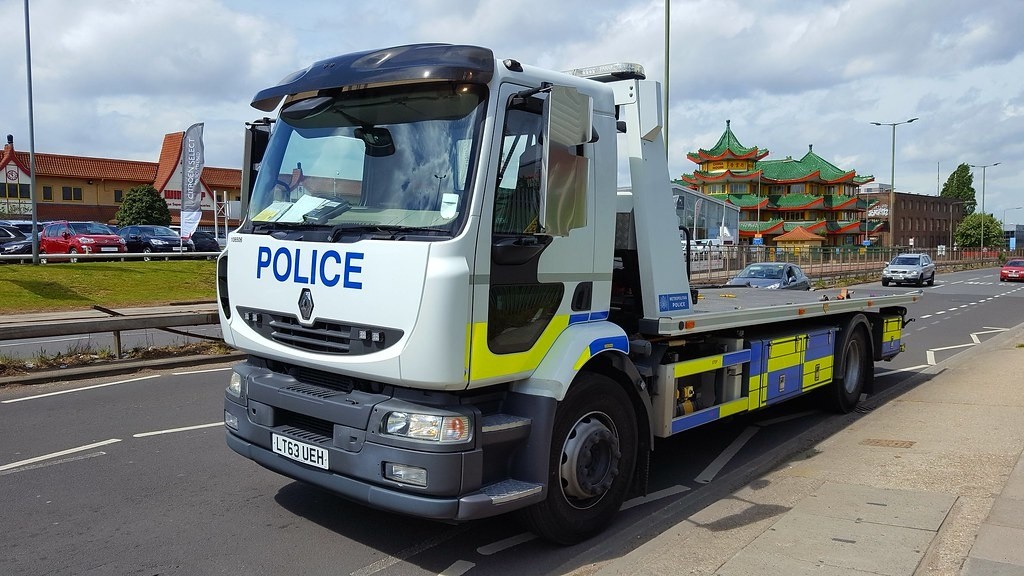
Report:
681,239,734,261
0,219,119,264
725,261,811,291
998,259,1024,282
183,231,221,253
116,225,193,262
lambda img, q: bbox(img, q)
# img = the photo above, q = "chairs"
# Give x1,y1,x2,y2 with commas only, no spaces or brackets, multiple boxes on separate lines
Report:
502,144,576,231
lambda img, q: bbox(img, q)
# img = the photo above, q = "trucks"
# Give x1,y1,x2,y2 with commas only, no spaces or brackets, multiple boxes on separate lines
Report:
210,39,930,548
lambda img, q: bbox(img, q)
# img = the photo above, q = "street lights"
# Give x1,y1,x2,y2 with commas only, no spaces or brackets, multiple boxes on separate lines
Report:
949,200,975,267
1002,206,1023,243
756,158,794,265
968,162,1002,266
870,117,920,264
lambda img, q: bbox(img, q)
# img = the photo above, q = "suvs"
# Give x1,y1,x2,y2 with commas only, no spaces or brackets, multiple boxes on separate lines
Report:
881,251,936,287
41,220,126,262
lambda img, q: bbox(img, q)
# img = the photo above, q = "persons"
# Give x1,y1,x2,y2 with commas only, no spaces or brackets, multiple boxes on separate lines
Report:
776,269,783,278
